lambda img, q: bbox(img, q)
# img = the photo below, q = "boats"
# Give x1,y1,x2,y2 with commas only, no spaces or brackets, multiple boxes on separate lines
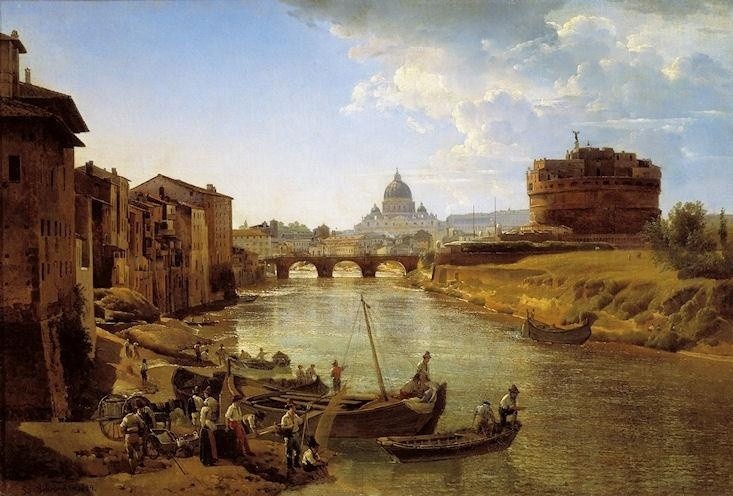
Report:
521,318,591,345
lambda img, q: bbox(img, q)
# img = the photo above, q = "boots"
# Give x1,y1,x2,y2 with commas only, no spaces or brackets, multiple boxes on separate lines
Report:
286,455,301,475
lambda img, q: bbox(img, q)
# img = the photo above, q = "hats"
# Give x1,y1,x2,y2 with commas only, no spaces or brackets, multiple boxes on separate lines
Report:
423,351,432,358
203,386,212,395
232,395,243,402
298,360,338,368
308,435,320,446
284,397,299,407
509,384,520,394
124,398,147,410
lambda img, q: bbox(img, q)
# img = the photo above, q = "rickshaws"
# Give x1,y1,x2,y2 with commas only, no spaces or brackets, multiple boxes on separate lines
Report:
146,428,188,475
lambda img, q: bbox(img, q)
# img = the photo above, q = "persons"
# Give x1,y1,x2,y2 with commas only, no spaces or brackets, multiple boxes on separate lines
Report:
117,337,440,476
496,384,528,437
470,400,497,439
